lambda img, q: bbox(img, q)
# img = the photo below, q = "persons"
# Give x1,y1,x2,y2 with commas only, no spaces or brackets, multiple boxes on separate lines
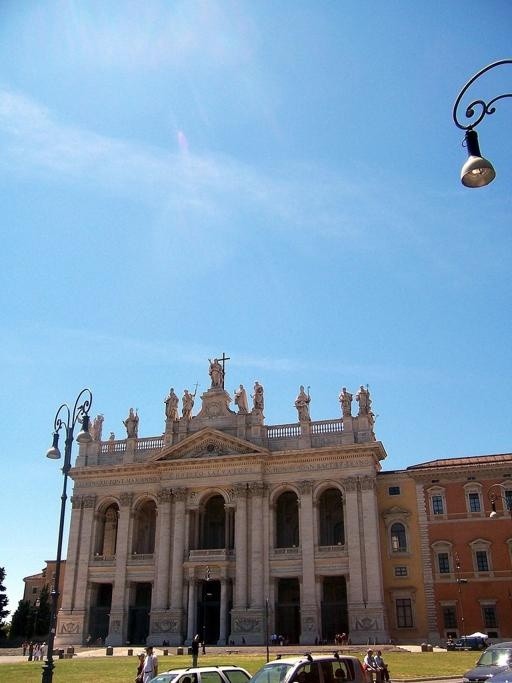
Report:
135,645,158,683
230,639,234,647
86,634,92,647
335,668,346,680
163,639,166,646
293,671,313,683
21,640,46,661
335,633,346,646
182,677,199,683
81,351,373,441
242,636,246,645
270,632,289,646
192,634,199,667
363,648,391,683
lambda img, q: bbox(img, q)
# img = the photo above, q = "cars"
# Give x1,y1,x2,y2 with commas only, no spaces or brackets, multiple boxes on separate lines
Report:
149,665,252,683
456,637,512,683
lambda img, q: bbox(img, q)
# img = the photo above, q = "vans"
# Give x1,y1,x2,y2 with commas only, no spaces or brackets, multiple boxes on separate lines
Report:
248,655,371,682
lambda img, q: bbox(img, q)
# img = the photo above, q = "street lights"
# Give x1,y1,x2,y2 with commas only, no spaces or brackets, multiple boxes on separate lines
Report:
452,552,467,635
41,389,93,683
453,57,511,188
201,565,211,655
487,484,512,520
